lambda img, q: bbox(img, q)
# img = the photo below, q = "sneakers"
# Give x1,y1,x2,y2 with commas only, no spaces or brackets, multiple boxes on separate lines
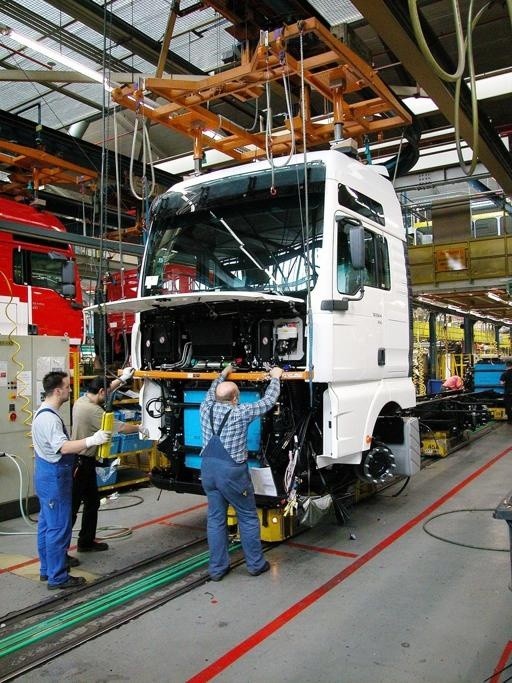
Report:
40,564,70,581
77,541,108,551
48,576,86,589
67,555,79,567
252,561,269,576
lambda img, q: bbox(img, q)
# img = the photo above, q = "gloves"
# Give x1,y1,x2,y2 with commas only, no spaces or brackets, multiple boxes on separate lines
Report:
138,425,151,438
115,367,136,383
86,429,112,449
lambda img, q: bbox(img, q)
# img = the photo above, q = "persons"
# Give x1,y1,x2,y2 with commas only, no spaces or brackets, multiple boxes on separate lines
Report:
439,374,465,392
30,370,112,590
69,366,150,550
198,364,284,582
498,360,511,425
